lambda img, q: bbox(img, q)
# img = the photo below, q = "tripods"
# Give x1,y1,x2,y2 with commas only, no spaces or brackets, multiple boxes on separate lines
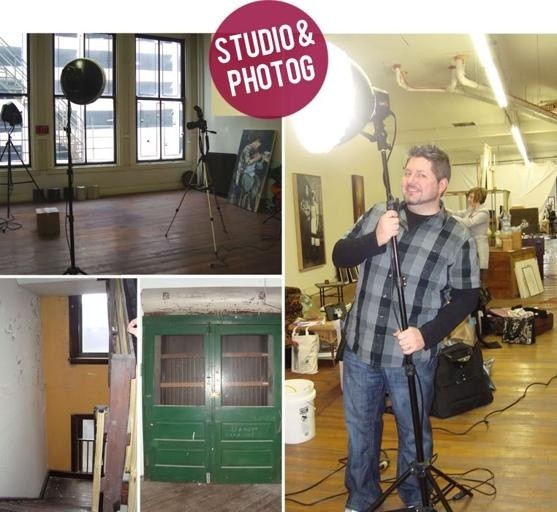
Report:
362,147,473,512
165,129,227,254
0,135,47,218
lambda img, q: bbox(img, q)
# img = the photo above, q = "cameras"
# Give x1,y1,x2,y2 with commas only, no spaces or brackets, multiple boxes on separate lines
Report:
187,105,207,132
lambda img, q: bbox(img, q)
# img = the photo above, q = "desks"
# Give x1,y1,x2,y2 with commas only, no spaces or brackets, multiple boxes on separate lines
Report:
480,247,538,299
522,233,557,279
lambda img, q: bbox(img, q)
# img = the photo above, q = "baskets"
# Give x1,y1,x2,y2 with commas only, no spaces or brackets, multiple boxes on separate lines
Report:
488,305,553,336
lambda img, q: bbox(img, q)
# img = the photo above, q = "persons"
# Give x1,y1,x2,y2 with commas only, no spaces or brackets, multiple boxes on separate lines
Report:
331,145,480,512
299,183,311,263
232,137,263,206
308,191,321,266
445,186,491,279
241,151,272,211
546,203,556,238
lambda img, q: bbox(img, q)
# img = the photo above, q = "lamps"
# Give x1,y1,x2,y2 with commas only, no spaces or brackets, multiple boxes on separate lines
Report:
285,40,473,512
61,59,106,275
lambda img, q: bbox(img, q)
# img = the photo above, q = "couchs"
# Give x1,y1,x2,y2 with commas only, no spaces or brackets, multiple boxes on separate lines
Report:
285,281,358,368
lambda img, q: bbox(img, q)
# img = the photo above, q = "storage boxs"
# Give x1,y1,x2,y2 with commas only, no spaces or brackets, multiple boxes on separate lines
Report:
36,208,61,238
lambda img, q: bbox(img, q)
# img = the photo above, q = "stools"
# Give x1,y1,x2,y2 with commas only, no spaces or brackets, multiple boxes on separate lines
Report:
316,281,346,308
32,184,100,204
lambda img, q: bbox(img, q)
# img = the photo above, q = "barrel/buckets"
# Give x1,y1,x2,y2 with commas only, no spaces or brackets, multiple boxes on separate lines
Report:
285,379,317,445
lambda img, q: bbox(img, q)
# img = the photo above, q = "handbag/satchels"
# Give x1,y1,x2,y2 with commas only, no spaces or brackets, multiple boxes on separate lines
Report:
502,317,535,345
291,326,320,374
431,342,493,419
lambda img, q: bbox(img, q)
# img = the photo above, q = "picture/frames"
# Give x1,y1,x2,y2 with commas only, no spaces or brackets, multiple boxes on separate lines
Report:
292,172,326,273
348,265,360,283
336,265,350,285
